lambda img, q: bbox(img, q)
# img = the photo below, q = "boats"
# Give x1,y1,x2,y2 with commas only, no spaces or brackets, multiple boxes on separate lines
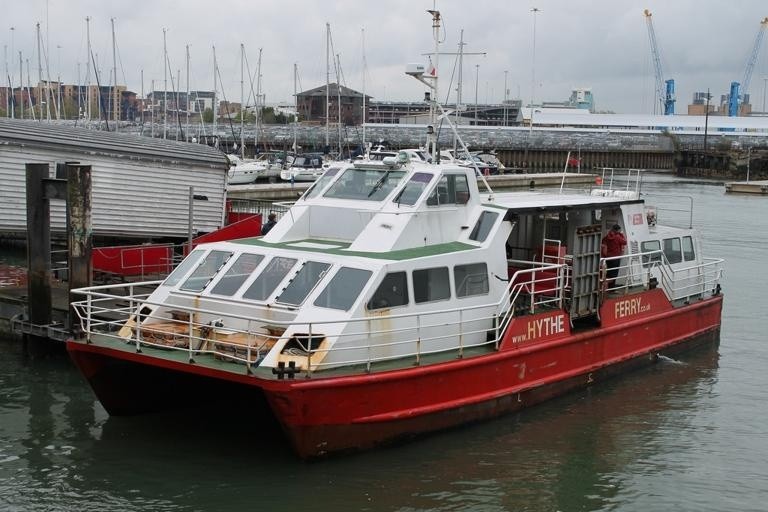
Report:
56,0,726,465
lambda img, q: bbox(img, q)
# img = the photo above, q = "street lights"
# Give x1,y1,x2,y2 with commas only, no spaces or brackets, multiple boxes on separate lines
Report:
524,4,542,138
187,184,207,257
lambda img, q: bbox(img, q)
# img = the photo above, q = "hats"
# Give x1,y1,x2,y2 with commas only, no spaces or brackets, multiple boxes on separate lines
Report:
613,224,620,231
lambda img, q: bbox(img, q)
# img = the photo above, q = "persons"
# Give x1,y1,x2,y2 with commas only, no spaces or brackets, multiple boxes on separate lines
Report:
602,224,627,294
261,214,277,235
